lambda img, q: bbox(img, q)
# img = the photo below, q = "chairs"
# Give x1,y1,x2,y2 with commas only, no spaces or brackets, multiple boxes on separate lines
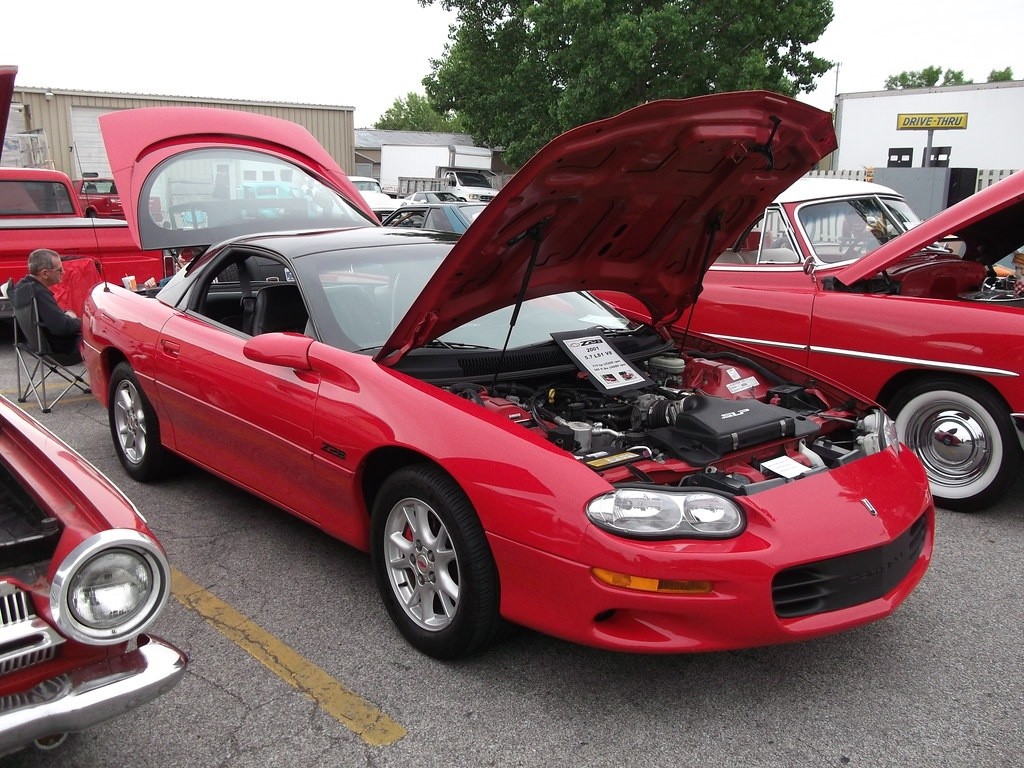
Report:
238,282,308,336
303,286,384,352
0,277,92,413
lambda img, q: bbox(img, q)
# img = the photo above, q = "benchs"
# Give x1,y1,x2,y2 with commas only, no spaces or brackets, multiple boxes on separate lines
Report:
714,247,798,263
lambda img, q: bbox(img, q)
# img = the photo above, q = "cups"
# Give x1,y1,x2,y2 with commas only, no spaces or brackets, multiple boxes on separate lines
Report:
122,276,137,292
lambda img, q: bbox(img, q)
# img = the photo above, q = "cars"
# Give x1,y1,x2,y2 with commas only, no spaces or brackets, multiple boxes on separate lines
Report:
1,392,187,764
0,161,1024,514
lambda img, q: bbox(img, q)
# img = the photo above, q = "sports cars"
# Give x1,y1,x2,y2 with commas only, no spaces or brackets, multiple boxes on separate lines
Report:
77,87,938,666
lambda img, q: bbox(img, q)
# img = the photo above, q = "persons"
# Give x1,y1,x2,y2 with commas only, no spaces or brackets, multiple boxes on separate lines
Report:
16,248,84,366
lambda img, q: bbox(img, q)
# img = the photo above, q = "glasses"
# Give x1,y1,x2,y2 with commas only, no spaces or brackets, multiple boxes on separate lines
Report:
41,266,62,272
